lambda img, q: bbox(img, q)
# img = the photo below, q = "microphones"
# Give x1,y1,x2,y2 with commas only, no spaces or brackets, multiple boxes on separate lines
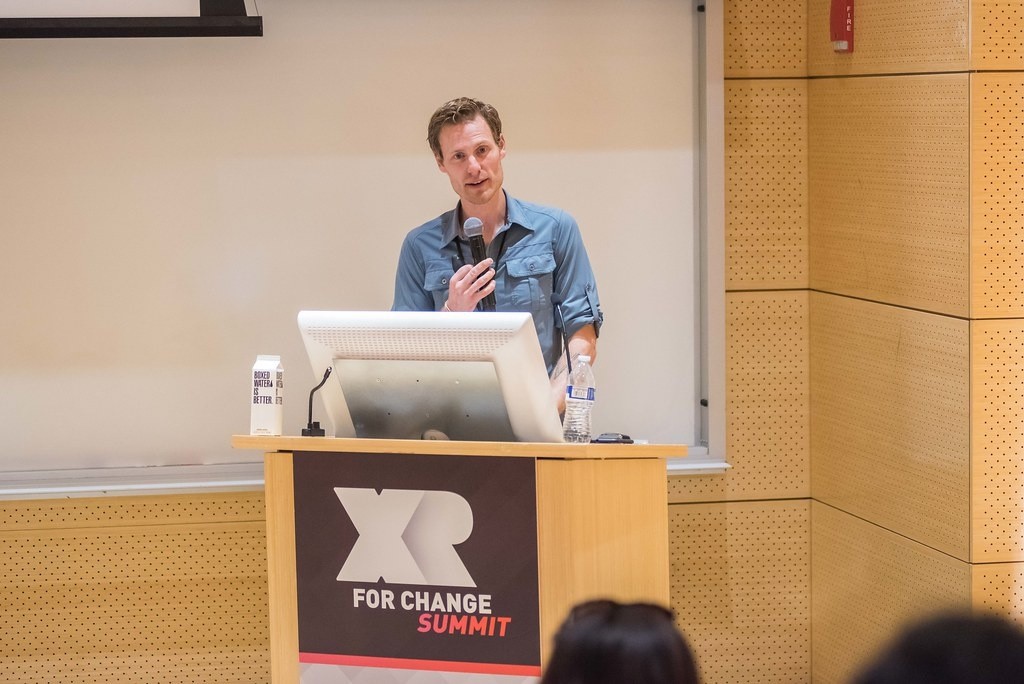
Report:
551,292,572,373
463,217,497,312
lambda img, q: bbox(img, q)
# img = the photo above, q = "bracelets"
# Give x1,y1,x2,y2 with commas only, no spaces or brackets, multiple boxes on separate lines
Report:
444,299,451,312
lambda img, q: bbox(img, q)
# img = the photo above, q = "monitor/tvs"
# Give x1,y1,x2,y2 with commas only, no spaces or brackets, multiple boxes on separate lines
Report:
297,311,565,444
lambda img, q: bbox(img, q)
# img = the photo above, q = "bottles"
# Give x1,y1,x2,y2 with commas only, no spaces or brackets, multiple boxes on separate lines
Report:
562,355,596,444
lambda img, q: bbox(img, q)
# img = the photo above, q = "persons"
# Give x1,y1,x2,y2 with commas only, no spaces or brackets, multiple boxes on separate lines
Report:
390,98,603,424
844,610,1024,684
540,600,697,684
